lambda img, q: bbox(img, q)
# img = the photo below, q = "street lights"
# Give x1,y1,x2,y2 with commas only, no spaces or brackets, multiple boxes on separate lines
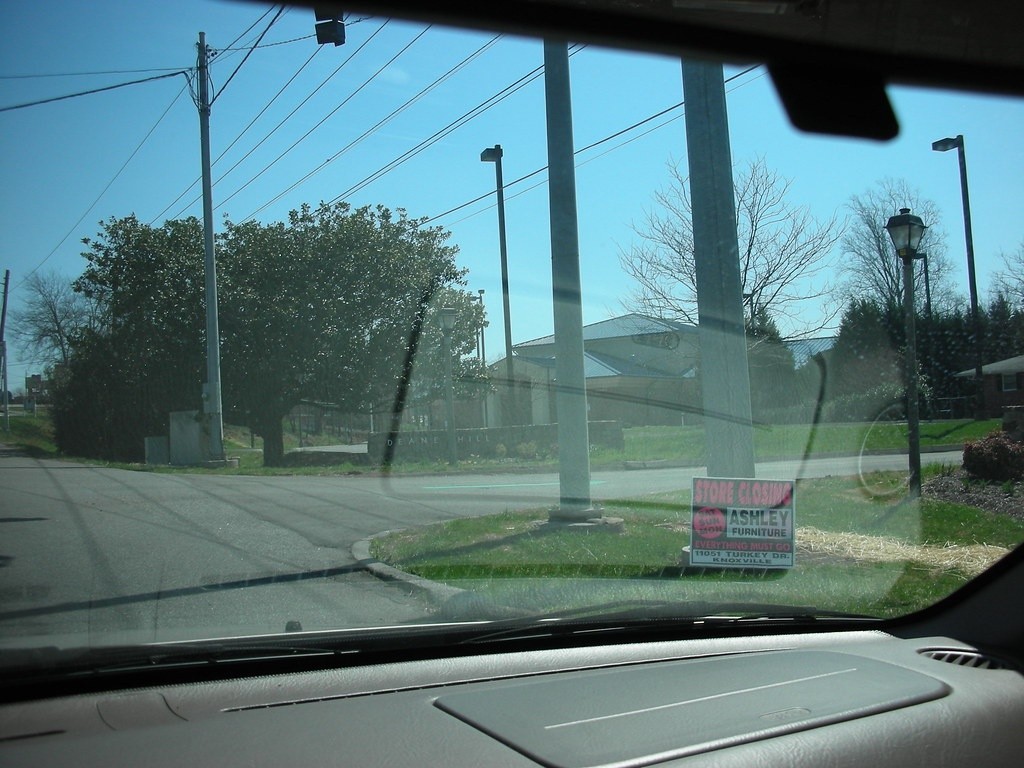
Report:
481,144,519,424
932,135,982,375
884,208,926,496
